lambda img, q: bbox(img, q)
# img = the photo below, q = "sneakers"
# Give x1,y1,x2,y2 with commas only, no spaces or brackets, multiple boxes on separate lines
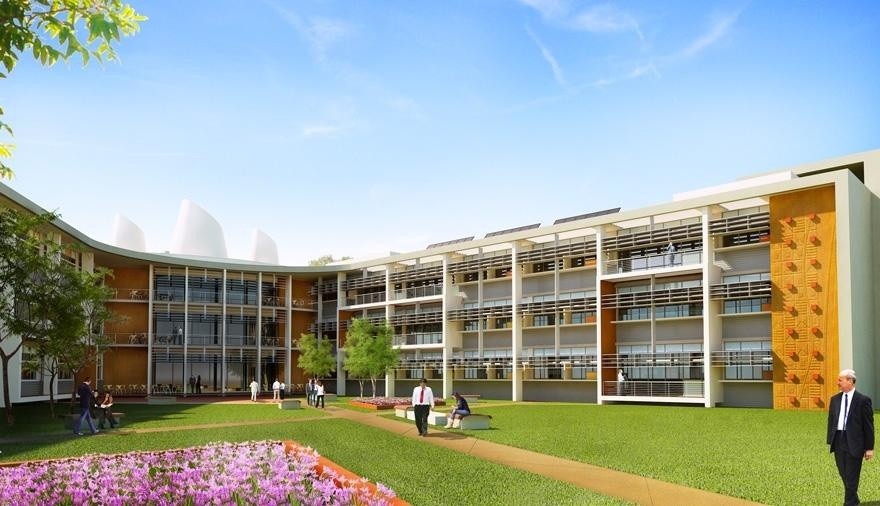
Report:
72,422,119,437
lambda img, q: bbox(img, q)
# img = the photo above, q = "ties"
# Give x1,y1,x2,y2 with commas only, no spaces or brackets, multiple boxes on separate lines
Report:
420,389,424,404
841,393,850,432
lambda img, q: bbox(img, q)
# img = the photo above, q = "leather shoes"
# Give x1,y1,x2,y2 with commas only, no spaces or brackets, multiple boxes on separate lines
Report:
416,428,428,438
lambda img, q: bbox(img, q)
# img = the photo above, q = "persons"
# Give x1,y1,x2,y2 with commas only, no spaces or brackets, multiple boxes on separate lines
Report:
272,377,281,399
89,389,119,428
189,374,196,394
194,374,201,394
280,381,285,399
443,392,470,429
617,369,625,396
827,370,874,505
411,379,435,438
248,379,259,401
171,325,183,345
73,376,99,436
306,378,325,409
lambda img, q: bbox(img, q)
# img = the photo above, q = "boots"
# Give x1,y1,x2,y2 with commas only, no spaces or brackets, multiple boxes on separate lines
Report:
444,418,461,430
